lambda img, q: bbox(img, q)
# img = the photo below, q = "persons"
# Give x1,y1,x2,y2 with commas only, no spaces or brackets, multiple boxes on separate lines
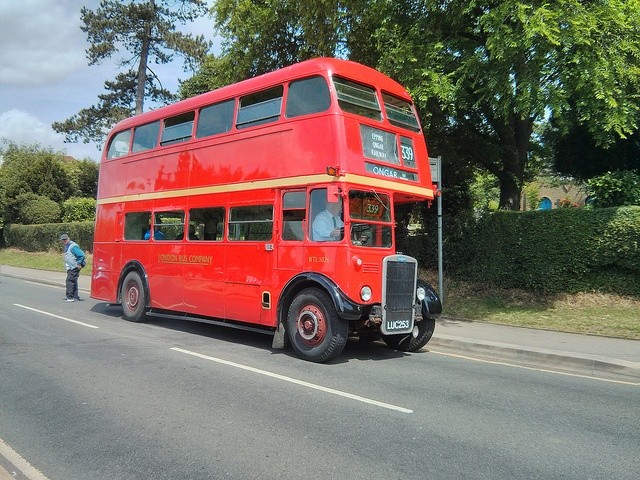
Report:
61,234,86,302
313,197,345,242
145,218,167,240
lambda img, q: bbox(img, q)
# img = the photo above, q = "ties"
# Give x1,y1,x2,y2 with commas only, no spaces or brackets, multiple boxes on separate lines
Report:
333,217,336,228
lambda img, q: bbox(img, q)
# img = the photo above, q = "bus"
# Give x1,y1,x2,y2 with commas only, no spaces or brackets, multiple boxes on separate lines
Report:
90,58,442,363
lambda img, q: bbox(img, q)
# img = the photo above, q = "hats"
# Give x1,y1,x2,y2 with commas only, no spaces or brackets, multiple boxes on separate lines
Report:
60,234,68,239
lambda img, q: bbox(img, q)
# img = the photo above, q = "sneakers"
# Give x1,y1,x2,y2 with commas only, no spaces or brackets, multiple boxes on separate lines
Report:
64,297,78,302
